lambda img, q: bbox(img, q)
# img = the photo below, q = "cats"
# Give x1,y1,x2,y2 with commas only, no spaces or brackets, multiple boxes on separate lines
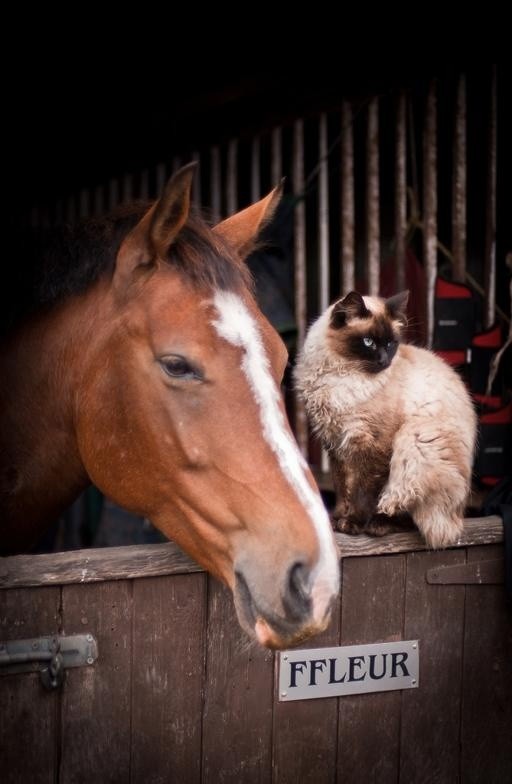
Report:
287,291,483,552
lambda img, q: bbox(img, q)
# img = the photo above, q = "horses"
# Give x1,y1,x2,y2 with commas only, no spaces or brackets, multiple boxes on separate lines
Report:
1,158,353,660
